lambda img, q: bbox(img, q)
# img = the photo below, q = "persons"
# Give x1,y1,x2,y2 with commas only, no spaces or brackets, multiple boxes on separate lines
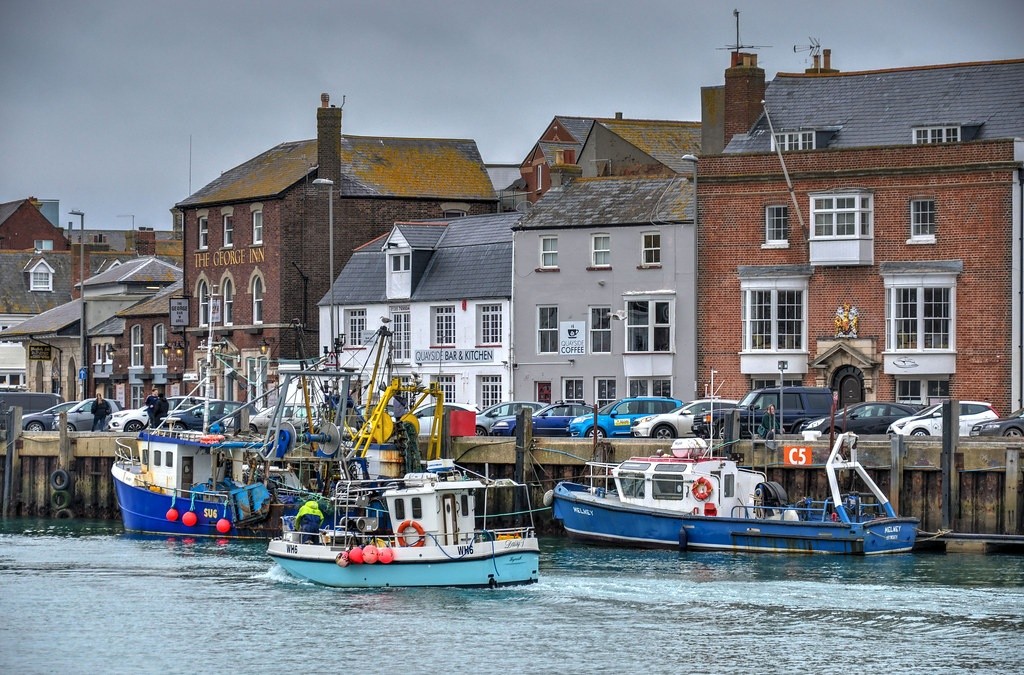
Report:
761,404,785,440
153,393,169,428
393,391,405,434
294,499,324,544
145,388,159,422
91,393,110,432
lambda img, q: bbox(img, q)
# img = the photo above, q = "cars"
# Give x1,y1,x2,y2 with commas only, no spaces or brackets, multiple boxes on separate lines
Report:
475,402,554,436
108,395,229,432
969,408,1024,439
885,401,1000,437
368,403,482,436
337,404,395,437
164,401,260,435
629,399,741,439
226,402,324,437
21,398,122,432
800,401,919,435
565,396,685,438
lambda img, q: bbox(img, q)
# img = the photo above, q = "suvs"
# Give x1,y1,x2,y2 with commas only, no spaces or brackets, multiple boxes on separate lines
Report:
691,385,837,440
488,400,600,437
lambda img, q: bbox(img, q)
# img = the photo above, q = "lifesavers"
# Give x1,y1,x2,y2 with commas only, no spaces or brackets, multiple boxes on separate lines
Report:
199,435,224,444
692,478,711,500
49,469,75,517
397,519,425,547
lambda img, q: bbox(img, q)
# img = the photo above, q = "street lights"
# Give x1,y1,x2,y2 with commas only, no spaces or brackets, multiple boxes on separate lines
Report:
69,210,86,401
310,177,333,413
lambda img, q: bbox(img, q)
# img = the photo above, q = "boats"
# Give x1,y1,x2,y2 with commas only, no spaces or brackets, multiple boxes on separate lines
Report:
266,319,540,589
545,364,922,556
108,287,317,541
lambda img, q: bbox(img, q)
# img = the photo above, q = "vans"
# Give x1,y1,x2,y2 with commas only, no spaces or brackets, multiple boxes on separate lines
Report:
0,392,67,429
